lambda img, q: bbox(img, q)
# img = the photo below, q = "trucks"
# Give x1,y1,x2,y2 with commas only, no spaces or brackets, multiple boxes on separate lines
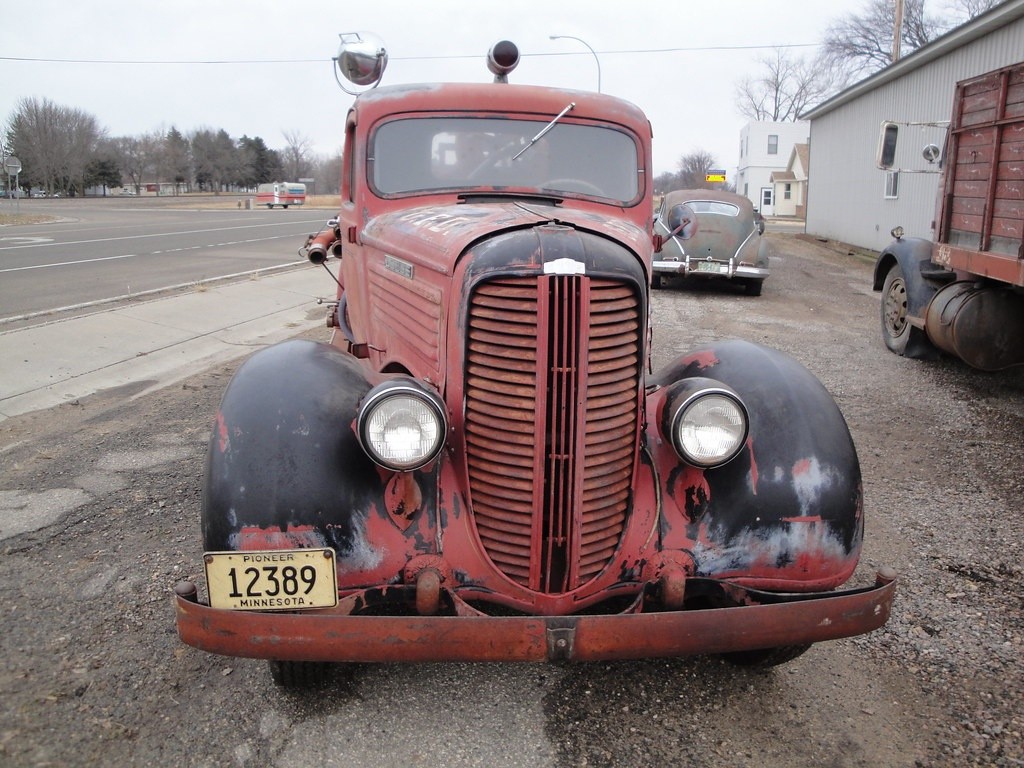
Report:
173,31,898,675
869,57,1024,370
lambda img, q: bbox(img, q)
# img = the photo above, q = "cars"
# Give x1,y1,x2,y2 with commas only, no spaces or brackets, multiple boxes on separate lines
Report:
120,191,136,195
35,191,58,197
651,189,773,295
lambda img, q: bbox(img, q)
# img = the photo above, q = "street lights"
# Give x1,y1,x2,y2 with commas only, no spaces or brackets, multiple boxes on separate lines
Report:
549,35,601,92
